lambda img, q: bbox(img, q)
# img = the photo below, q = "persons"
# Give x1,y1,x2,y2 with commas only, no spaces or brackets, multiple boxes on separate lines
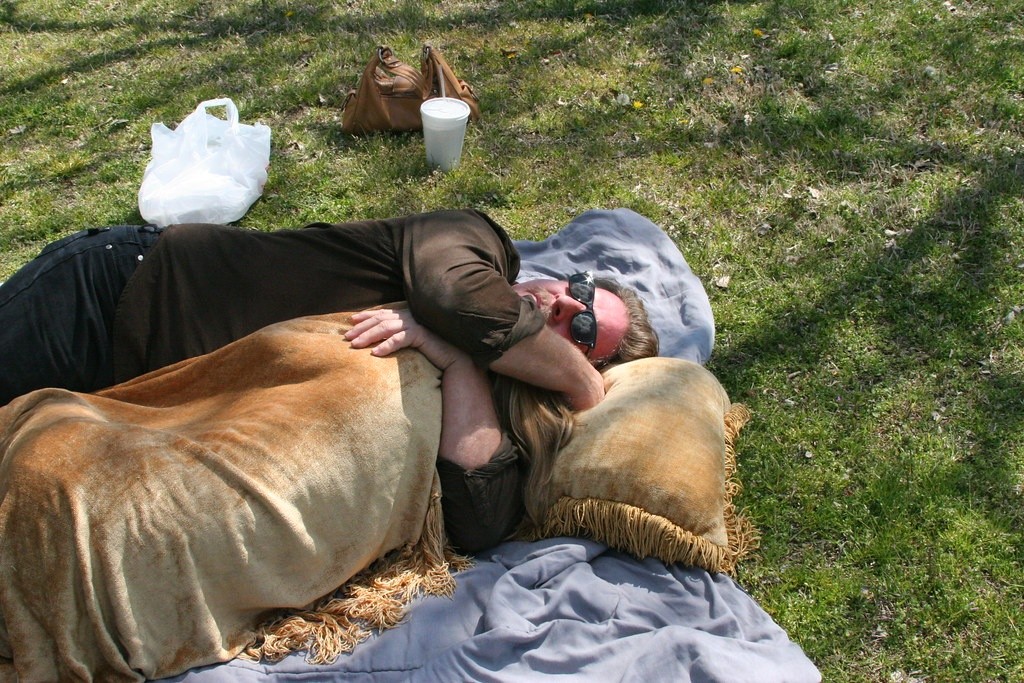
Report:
1,305,582,682
1,207,660,560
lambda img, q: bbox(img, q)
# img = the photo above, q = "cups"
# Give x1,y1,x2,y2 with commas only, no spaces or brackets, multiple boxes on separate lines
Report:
420,98,470,171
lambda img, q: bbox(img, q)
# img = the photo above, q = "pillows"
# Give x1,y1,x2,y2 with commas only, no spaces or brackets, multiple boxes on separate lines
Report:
516,351,764,572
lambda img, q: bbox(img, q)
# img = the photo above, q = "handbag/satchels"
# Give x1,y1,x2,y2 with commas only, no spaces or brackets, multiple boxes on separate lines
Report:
342,39,481,135
137,97,271,228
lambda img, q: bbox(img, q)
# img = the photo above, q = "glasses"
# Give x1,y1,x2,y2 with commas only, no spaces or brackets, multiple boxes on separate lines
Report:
568,271,597,356
